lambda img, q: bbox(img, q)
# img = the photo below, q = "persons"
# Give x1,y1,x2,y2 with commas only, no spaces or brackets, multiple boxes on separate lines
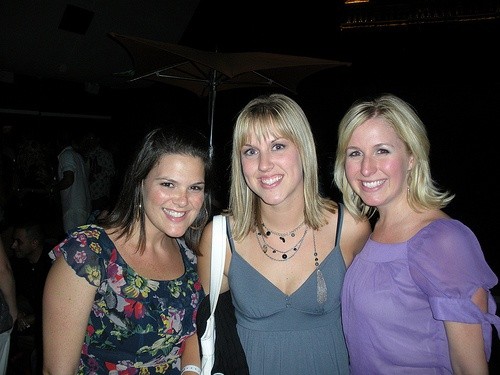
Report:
198,93,372,375
1,136,125,375
43,127,212,375
334,96,499,375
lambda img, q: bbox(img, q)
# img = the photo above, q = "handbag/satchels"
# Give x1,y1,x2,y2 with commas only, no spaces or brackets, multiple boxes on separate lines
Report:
196,215,249,375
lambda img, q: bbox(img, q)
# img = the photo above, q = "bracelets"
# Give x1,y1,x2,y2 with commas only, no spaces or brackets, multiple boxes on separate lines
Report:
182,364,200,374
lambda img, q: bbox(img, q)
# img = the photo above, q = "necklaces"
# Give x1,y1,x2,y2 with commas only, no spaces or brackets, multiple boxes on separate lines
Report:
250,199,328,305
